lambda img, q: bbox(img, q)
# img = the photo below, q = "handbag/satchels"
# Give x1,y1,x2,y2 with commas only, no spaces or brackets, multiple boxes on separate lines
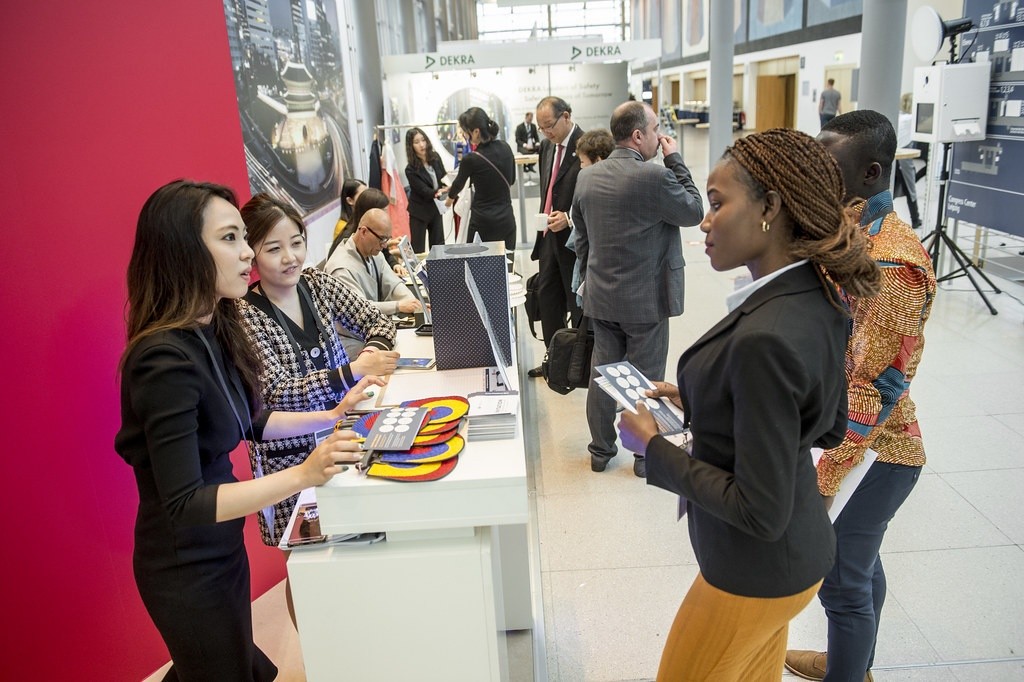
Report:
524,272,571,341
542,313,594,395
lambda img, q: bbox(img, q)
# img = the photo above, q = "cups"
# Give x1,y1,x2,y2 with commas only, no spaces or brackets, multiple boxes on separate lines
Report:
533,213,553,231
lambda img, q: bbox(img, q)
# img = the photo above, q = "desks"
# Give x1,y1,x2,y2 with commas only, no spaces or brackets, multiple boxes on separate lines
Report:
676,119,699,160
515,155,539,250
696,123,739,129
895,149,921,160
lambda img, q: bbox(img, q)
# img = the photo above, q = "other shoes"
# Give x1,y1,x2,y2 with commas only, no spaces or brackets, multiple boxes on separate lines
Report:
912,219,923,230
523,165,537,174
528,365,544,377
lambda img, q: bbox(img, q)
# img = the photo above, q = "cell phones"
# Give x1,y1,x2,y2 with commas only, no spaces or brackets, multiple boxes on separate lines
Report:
395,358,436,370
287,503,326,547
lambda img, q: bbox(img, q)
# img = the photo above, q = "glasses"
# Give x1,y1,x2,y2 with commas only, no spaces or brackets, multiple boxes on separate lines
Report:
538,113,563,133
358,227,392,243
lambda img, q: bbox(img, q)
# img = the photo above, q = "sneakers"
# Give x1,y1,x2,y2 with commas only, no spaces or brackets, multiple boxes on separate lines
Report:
784,650,874,682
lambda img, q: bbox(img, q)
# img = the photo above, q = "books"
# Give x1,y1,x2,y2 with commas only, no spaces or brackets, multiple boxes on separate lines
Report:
277,486,385,550
463,390,518,442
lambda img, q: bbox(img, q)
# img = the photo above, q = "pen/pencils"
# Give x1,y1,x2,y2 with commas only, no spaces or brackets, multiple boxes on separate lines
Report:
616,405,625,413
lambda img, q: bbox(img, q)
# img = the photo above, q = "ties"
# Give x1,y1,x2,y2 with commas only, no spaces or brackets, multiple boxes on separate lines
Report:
527,125,529,130
543,144,565,237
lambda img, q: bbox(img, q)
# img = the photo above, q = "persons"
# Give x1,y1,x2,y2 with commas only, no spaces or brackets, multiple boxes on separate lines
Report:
819,78,842,130
112,180,388,682
515,112,541,173
324,180,422,363
405,127,451,254
528,96,583,378
234,193,400,634
784,109,937,682
617,128,885,682
569,101,705,477
444,108,516,273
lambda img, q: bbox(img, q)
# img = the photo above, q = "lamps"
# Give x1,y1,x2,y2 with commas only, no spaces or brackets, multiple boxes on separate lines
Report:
911,6,1001,315
